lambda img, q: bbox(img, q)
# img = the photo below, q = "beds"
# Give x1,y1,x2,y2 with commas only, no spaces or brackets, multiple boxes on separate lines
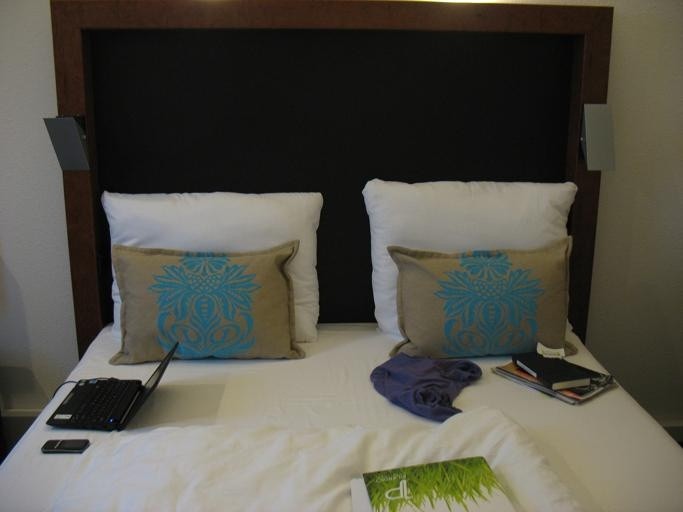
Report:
0,1,683,512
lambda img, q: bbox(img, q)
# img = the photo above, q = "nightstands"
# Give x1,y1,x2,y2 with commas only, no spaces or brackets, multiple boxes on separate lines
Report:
0,368,49,462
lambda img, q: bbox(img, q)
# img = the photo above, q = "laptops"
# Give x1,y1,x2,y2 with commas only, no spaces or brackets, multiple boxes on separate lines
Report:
46,342,179,431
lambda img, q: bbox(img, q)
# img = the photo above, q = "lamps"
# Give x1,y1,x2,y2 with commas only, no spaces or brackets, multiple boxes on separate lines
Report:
580,104,617,171
43,117,92,171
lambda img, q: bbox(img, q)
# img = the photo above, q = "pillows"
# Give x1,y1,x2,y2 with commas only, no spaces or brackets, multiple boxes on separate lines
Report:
108,239,305,366
360,178,578,341
387,235,578,358
100,190,324,359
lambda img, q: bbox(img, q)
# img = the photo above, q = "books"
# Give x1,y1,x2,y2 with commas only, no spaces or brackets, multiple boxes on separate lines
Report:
348,453,518,512
491,347,617,406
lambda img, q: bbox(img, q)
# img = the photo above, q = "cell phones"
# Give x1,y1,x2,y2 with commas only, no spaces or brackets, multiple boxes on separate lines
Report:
41,439,90,454
